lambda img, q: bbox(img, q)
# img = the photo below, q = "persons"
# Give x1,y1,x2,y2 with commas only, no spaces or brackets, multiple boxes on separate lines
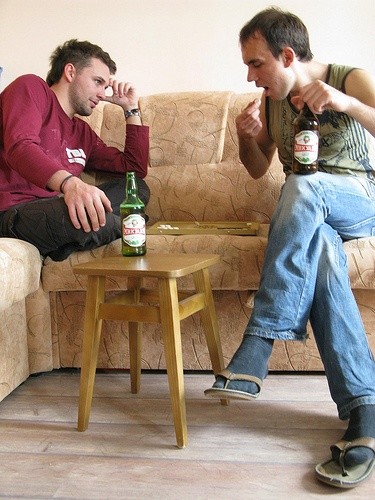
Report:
0,39,151,262
204,7,375,489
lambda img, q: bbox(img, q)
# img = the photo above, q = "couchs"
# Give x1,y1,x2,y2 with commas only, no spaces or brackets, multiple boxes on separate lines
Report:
0,91,375,402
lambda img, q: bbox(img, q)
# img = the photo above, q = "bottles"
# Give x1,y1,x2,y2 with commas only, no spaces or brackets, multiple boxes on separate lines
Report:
120,171,147,256
292,102,319,175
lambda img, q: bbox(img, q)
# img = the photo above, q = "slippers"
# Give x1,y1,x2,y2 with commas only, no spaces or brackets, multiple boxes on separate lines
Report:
203,369,263,401
314,437,375,488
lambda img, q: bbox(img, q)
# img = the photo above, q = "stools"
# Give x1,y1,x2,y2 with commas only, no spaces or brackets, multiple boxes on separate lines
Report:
73,252,230,448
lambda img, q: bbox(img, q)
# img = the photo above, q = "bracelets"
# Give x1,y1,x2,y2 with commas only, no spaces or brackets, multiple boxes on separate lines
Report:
124,109,140,119
59,174,77,193
